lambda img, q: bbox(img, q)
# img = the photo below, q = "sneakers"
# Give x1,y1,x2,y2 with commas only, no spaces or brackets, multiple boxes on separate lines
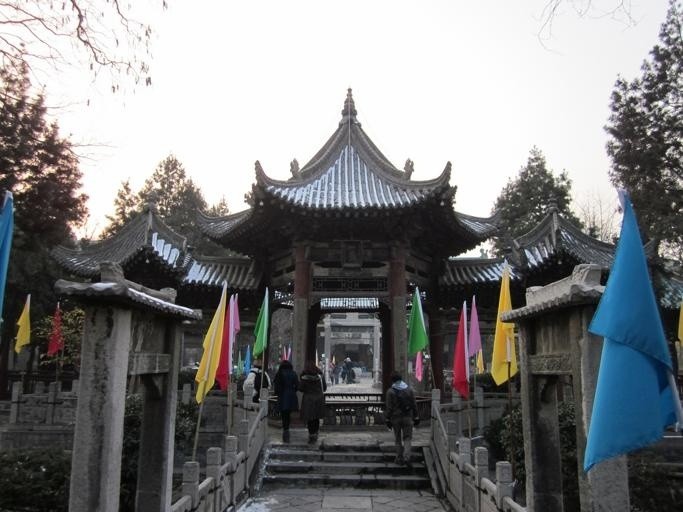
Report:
395,456,408,465
282,432,319,444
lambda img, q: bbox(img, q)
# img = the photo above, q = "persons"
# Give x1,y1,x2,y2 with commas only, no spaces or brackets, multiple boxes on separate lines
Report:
273,360,298,445
319,354,366,385
296,359,328,443
386,369,420,466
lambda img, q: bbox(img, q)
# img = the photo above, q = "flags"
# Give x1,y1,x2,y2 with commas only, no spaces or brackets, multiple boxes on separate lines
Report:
45,304,66,357
451,304,471,398
469,300,482,356
406,289,428,354
490,260,519,386
15,299,31,355
580,194,683,477
676,302,683,347
477,350,484,375
0,192,16,321
416,353,425,381
191,283,291,405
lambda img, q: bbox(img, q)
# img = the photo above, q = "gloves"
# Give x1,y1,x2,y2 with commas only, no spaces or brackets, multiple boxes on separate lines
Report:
385,420,393,431
413,417,421,426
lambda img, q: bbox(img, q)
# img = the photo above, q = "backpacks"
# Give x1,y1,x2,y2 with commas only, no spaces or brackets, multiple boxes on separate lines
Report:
250,370,268,392
